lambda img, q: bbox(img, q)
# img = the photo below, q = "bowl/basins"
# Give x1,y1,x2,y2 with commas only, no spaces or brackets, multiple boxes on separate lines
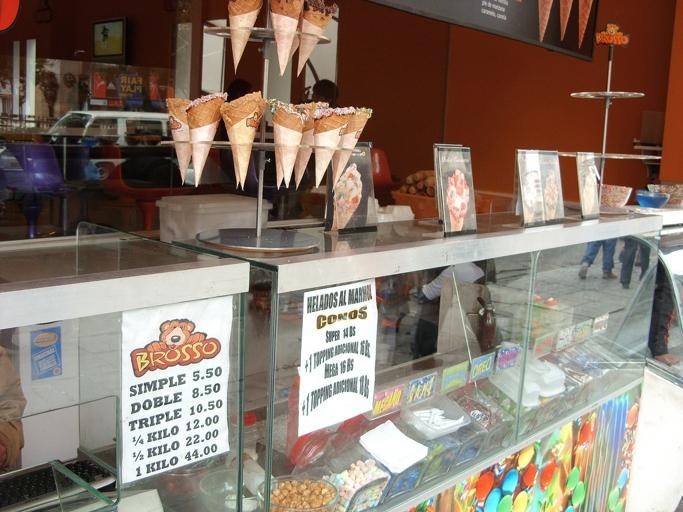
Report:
0,132,164,146
198,471,340,512
601,184,683,211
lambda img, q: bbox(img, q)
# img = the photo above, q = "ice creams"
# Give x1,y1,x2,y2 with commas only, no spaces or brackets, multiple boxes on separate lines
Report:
335,163,363,229
446,169,470,232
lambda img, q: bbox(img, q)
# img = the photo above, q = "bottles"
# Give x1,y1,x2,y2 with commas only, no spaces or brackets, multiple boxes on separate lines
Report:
228,412,258,461
230,453,278,496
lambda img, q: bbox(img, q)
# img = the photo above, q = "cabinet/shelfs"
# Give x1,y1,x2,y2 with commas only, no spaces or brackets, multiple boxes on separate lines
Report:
627,204,680,512
0,233,251,512
170,206,663,512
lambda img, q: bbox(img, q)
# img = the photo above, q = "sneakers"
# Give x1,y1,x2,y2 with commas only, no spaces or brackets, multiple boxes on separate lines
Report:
578,264,631,288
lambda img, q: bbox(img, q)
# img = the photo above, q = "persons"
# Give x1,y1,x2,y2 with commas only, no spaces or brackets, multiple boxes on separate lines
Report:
648,260,680,367
617,236,651,288
0,344,27,473
577,239,617,280
217,77,257,182
298,79,343,112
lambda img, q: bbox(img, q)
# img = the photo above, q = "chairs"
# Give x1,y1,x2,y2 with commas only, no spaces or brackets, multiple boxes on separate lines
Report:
1,141,194,236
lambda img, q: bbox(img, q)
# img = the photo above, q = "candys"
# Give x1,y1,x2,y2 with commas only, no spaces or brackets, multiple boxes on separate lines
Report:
323,458,383,512
407,403,638,512
